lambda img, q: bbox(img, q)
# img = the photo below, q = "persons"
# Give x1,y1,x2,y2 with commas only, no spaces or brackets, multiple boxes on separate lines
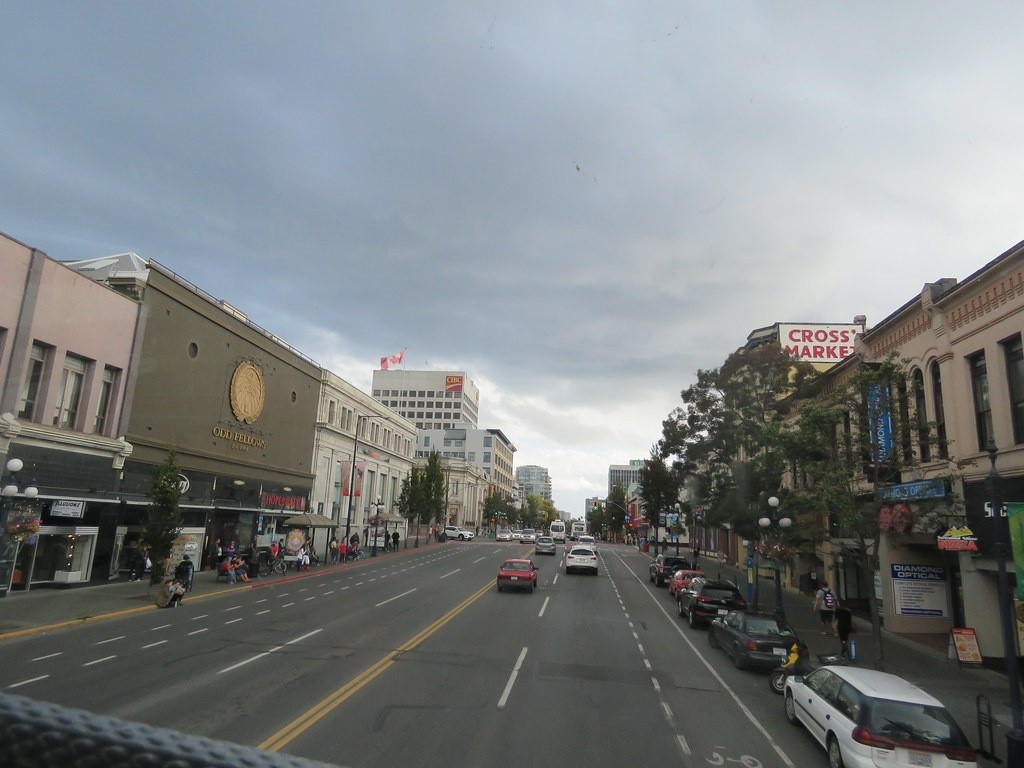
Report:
155,578,185,608
265,538,287,575
211,538,220,570
221,554,253,585
717,547,728,569
429,525,438,540
329,531,360,564
386,529,399,552
834,600,852,662
296,537,321,572
128,544,150,582
812,581,840,635
226,540,235,564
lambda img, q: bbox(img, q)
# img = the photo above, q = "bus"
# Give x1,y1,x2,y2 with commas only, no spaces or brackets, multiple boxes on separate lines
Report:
570,521,587,541
549,519,566,545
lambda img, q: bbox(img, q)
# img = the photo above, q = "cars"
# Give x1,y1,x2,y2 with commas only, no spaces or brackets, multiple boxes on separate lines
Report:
782,665,977,768
441,525,475,542
708,609,810,672
566,535,599,575
534,536,557,555
497,559,539,592
495,526,546,545
649,553,748,627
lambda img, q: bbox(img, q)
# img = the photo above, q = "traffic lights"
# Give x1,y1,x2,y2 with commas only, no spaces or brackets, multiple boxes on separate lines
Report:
629,524,633,527
593,501,598,510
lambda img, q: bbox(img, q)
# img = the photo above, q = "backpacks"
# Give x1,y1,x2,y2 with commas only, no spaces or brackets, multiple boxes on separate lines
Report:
821,589,834,608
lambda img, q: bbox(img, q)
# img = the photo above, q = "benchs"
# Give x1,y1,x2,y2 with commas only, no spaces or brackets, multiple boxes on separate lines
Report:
214,561,250,583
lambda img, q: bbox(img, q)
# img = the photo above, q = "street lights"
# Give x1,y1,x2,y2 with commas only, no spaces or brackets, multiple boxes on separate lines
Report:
671,501,681,555
346,415,390,536
759,496,793,631
0,457,37,544
369,495,386,558
444,463,464,542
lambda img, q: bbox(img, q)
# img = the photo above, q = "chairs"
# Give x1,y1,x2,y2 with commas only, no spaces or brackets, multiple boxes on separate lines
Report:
175,564,195,593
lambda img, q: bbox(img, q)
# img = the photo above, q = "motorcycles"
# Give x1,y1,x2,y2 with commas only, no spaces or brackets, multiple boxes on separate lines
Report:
770,640,846,695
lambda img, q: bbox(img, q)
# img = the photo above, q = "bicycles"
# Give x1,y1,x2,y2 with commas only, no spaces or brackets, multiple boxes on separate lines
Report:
262,559,288,577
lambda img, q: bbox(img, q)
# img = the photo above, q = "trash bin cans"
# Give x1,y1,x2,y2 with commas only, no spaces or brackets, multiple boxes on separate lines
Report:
488,531,494,539
641,542,649,552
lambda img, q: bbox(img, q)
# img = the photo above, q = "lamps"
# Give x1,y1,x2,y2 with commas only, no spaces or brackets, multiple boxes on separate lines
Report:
283,486,292,492
232,479,246,486
